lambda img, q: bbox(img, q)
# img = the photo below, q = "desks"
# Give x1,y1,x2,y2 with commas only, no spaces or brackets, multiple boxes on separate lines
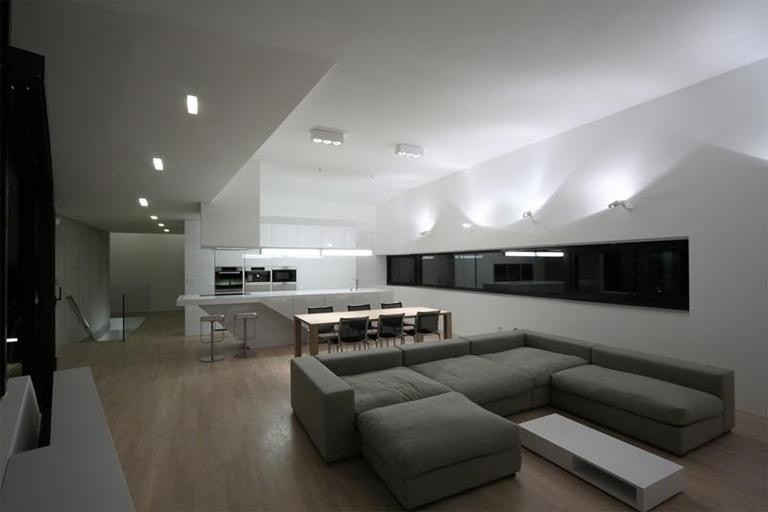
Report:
1,366,134,512
295,306,452,354
176,287,392,348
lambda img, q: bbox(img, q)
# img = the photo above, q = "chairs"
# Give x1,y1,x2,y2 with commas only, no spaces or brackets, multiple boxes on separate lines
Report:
308,303,441,352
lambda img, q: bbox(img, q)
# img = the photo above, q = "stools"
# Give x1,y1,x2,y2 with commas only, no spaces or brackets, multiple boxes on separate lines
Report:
199,312,256,361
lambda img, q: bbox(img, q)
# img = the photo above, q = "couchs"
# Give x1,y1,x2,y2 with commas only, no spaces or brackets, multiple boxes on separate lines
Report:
291,329,735,508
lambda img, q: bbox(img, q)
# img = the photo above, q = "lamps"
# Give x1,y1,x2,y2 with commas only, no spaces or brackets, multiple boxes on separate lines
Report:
310,129,344,146
608,199,635,211
395,143,424,158
